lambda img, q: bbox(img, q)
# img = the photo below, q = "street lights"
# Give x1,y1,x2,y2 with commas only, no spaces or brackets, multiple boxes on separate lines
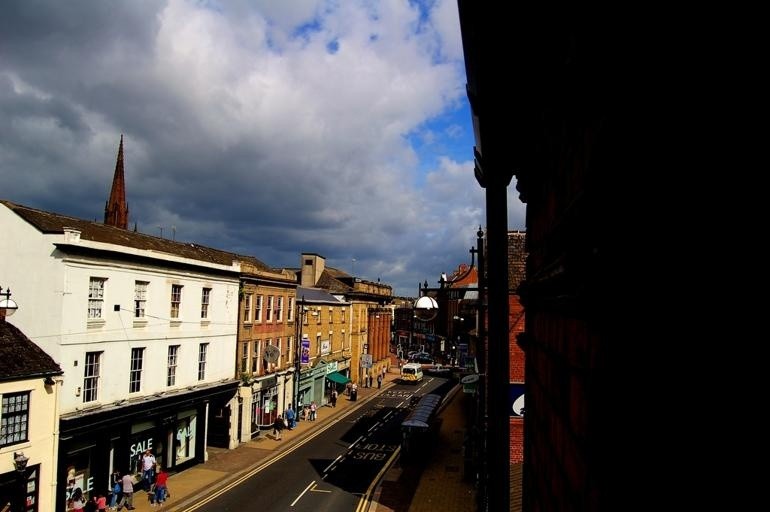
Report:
0,285,19,317
413,225,487,511
296,294,318,421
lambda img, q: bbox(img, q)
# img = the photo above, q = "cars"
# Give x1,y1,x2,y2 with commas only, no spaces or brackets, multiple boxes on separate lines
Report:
390,343,450,375
452,368,469,381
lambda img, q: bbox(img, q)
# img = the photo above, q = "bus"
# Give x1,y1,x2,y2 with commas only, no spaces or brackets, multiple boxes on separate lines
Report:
401,363,423,386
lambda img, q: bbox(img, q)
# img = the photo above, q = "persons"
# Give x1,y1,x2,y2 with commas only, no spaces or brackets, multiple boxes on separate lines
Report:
273,365,387,440
70,450,168,512
